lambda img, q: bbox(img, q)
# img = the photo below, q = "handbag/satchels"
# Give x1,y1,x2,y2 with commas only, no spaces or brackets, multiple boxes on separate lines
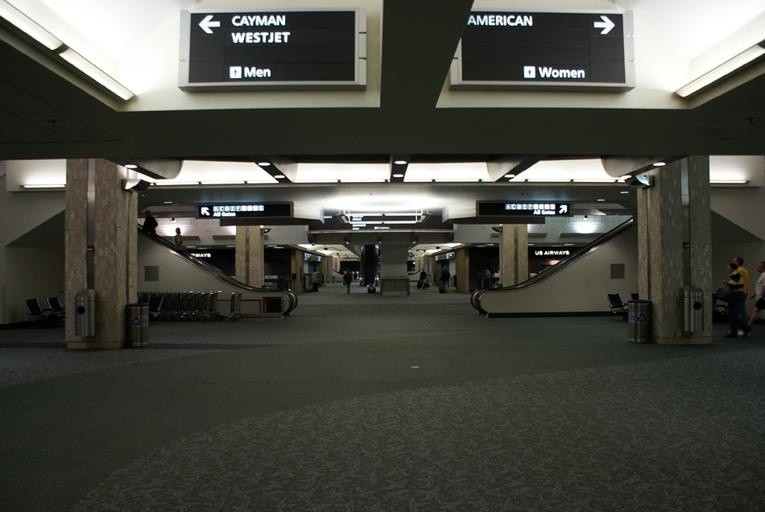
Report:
716,284,739,305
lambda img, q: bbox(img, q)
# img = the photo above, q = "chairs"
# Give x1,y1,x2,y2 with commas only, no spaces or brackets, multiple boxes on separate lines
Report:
46,296,65,325
608,293,630,321
20,299,57,326
631,294,638,300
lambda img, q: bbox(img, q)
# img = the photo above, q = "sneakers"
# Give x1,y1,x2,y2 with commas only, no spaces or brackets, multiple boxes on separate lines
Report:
724,326,752,338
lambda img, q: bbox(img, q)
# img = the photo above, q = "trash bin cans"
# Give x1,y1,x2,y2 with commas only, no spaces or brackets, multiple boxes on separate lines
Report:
627,301,650,344
684,287,705,333
128,303,149,346
75,289,96,337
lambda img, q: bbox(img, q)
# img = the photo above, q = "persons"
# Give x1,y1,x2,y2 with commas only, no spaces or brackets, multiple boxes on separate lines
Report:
342,270,352,295
746,261,765,328
172,228,186,254
721,261,752,338
439,266,450,292
140,209,158,237
418,267,427,289
728,256,750,331
